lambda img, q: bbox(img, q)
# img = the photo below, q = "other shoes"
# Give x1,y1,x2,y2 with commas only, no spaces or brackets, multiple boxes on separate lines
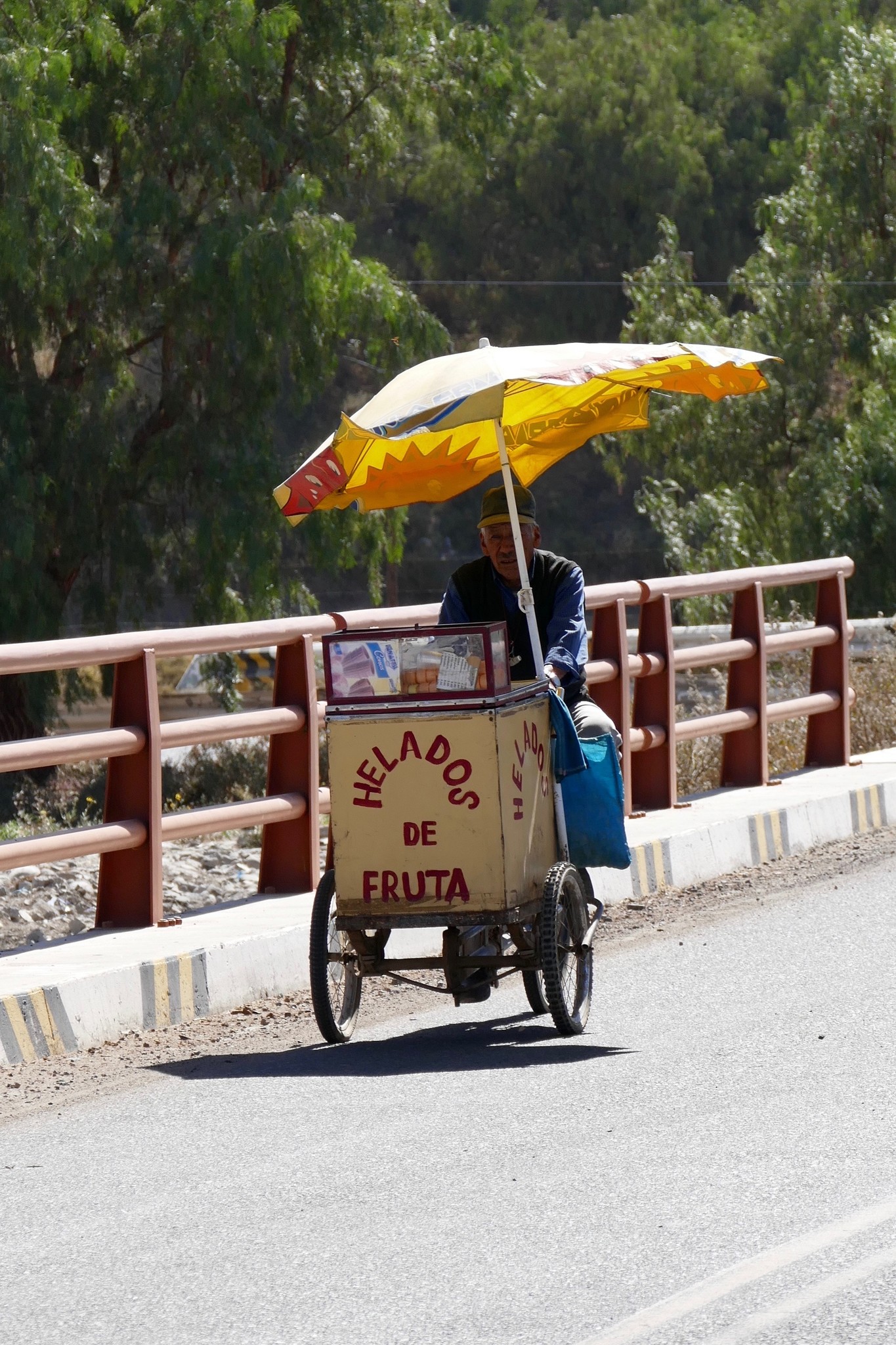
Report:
459,968,491,1004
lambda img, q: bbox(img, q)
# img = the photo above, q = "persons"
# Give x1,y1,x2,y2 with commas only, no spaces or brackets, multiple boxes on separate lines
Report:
436,485,622,1003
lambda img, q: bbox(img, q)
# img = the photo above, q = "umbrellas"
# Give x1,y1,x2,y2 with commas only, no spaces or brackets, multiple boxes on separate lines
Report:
272,336,783,682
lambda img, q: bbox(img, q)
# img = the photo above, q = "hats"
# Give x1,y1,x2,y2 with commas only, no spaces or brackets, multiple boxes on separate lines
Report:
477,485,536,529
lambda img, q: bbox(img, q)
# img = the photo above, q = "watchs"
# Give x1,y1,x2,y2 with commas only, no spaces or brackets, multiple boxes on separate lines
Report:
545,670,561,688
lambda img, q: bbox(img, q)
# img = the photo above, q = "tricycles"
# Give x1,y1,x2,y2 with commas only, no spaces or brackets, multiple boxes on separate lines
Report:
304,615,605,1045
